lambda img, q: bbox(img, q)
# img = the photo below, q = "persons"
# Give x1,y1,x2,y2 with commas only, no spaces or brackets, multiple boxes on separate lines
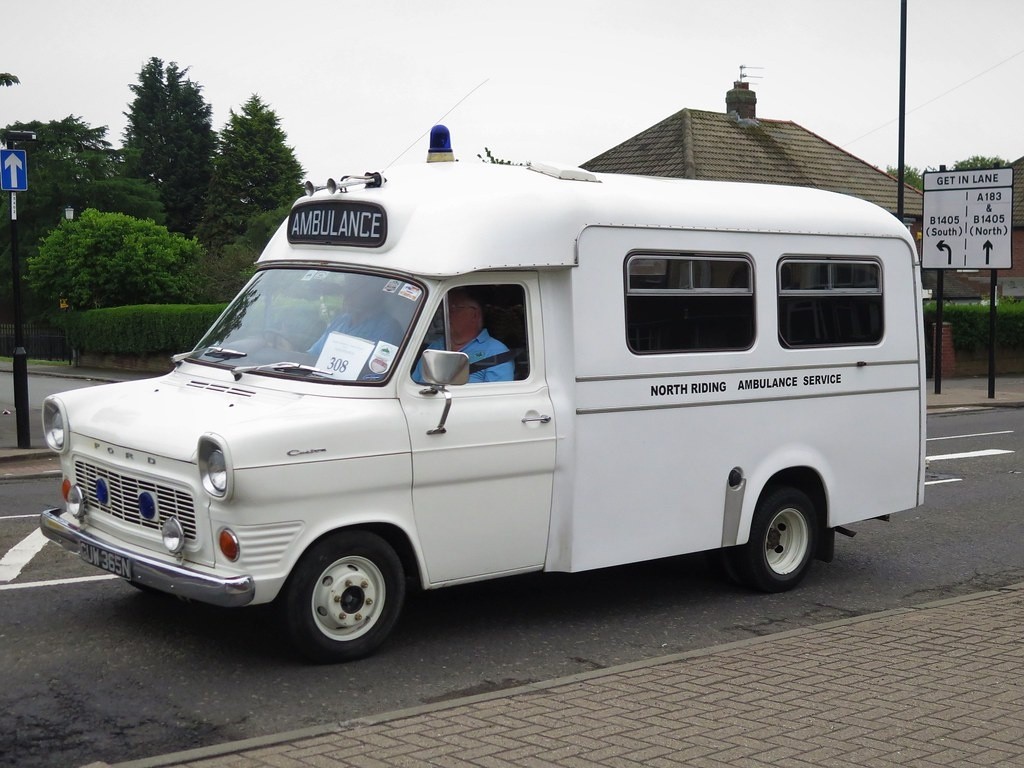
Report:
361,292,515,382
268,273,403,384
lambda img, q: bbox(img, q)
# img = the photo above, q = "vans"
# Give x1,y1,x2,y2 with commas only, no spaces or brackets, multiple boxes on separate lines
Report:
37,121,927,666
